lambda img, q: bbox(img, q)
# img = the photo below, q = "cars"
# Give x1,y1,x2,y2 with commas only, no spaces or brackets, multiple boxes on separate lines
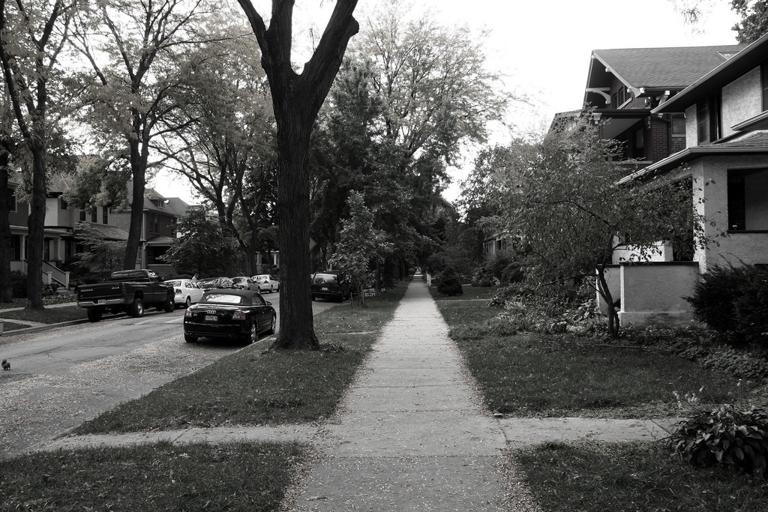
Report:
231,277,261,294
196,277,242,293
163,278,205,309
184,289,276,344
251,274,280,292
312,270,355,302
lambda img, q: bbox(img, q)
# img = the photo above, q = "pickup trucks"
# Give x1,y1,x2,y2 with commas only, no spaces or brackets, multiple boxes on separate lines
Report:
75,269,175,322
